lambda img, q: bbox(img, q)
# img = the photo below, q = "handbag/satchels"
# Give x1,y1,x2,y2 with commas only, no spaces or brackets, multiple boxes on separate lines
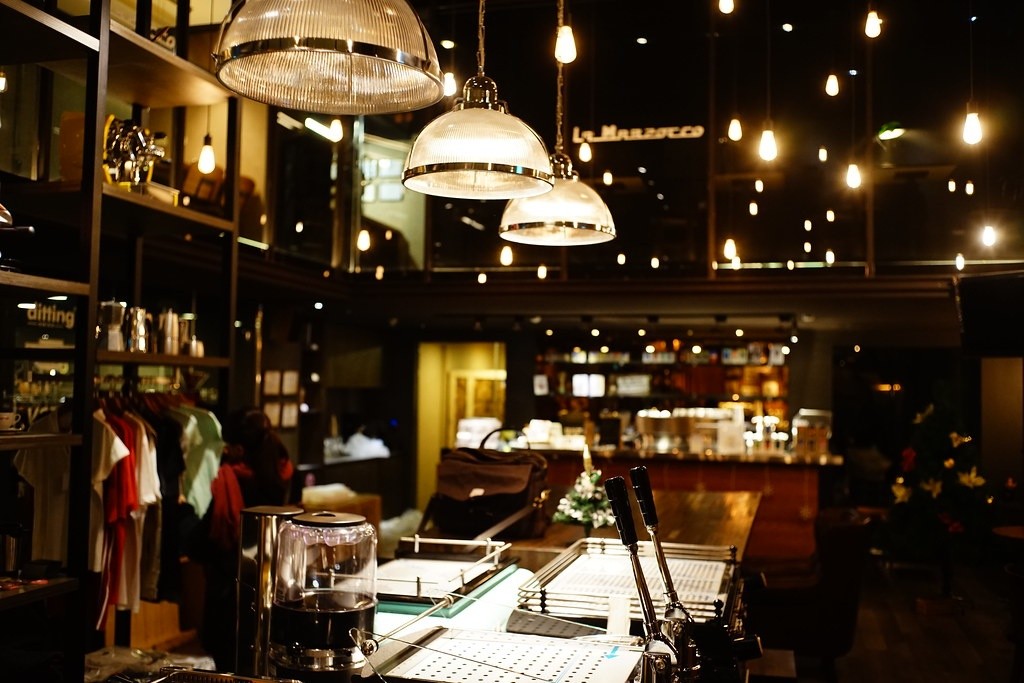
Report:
419,427,549,551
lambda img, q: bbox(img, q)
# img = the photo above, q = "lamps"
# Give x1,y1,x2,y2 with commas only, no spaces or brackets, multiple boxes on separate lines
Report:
198,104,216,173
211,0,445,115
400,0,556,201
498,0,617,246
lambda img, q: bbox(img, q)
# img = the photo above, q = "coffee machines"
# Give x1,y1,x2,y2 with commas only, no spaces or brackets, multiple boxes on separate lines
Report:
237,498,378,682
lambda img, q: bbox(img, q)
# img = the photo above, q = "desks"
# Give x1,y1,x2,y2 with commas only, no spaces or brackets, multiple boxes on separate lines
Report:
163,491,765,683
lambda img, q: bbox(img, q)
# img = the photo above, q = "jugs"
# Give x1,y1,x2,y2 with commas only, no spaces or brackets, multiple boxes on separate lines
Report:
156,307,179,356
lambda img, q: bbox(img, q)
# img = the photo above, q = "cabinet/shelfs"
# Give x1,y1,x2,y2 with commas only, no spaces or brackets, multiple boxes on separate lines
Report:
0,1,343,683
504,324,799,446
423,0,1024,278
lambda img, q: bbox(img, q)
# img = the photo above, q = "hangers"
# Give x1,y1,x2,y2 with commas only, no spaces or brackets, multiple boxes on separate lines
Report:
12,371,219,428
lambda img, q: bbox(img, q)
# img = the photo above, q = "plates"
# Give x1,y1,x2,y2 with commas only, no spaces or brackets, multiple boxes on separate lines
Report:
0,427,22,435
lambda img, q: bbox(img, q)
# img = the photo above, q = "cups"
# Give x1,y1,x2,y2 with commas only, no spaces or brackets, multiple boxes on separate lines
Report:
0,413,21,429
